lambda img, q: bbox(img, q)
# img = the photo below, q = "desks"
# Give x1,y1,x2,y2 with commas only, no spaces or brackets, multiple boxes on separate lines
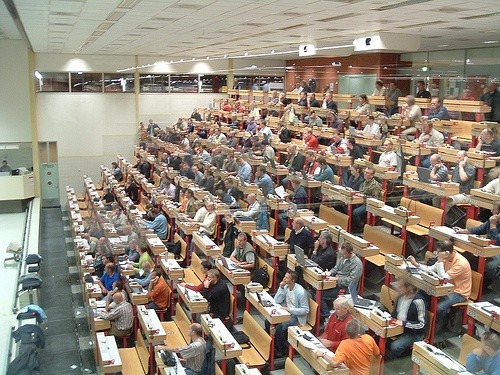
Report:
64,88,500,375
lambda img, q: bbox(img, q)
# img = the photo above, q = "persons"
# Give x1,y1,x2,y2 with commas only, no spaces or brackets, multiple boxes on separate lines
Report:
0,160,11,173
81,75,500,375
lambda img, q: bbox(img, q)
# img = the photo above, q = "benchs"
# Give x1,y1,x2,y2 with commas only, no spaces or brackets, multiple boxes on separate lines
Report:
379,285,434,348
182,252,205,287
284,357,305,375
76,185,86,202
424,251,483,333
172,232,187,266
80,201,92,220
78,193,89,210
299,298,319,331
160,302,193,348
234,238,275,295
257,216,276,248
381,196,444,253
457,333,484,367
235,310,273,371
279,227,292,261
315,204,349,236
161,223,171,243
363,223,404,278
118,329,150,375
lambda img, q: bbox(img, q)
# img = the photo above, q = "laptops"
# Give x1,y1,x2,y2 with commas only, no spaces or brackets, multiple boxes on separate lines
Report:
300,170,314,181
256,291,274,307
270,159,286,169
185,290,199,300
294,245,318,267
417,166,439,183
348,281,377,309
349,126,364,138
402,256,427,275
218,253,239,270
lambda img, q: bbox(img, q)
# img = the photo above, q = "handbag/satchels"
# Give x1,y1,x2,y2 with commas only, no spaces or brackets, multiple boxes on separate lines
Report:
164,242,175,252
172,240,181,255
231,331,249,344
252,264,269,289
